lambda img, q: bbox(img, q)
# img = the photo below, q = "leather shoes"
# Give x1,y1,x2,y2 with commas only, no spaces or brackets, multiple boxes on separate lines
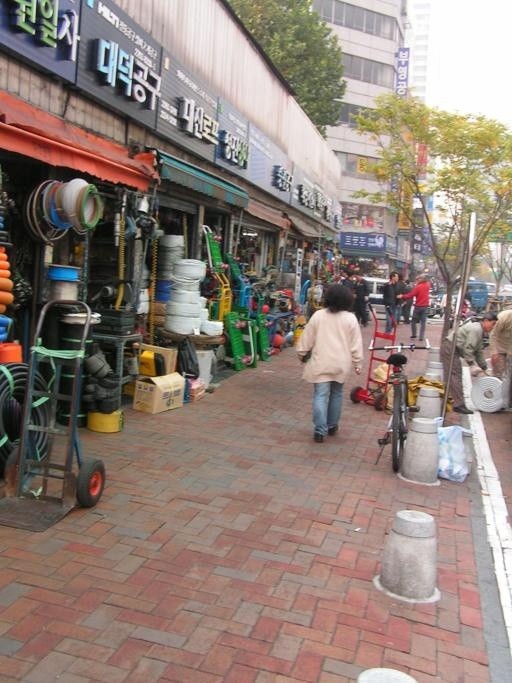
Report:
455,405,473,414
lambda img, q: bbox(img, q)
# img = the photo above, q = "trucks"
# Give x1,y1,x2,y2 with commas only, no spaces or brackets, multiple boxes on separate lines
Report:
465,281,488,312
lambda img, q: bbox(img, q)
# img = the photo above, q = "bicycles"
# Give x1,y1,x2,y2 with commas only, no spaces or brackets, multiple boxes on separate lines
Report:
367,336,432,473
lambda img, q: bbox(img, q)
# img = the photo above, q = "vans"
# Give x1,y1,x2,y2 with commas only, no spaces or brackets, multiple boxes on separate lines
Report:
484,283,496,297
351,277,415,322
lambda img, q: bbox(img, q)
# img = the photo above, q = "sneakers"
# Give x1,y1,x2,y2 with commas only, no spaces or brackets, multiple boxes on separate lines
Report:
315,432,325,442
327,425,338,435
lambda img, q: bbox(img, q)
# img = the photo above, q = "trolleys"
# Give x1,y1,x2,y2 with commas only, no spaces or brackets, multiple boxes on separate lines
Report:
0,300,105,531
350,303,398,411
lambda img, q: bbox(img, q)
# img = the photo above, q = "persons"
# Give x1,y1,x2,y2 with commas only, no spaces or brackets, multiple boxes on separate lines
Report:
489,308,512,380
343,271,357,298
441,311,499,415
396,273,407,324
396,274,433,341
300,265,318,324
402,280,413,324
383,271,401,334
295,284,364,443
353,270,369,327
